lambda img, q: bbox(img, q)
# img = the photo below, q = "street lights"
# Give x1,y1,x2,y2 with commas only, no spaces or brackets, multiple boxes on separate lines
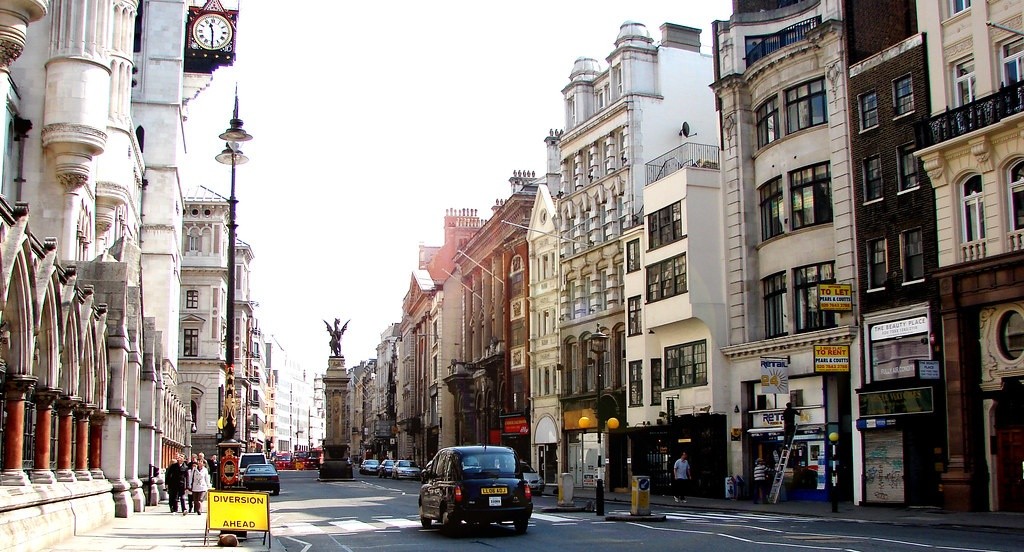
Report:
592,323,611,516
214,82,255,489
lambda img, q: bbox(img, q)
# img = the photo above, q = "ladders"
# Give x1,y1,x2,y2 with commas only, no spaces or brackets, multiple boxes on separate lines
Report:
767,414,800,504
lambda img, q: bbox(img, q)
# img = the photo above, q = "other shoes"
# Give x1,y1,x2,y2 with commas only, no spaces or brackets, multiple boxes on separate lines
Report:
197,511,201,515
785,444,789,450
189,505,193,513
673,496,679,503
183,510,188,516
681,500,687,503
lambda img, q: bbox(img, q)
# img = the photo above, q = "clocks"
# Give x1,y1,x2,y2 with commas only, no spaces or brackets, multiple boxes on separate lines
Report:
193,14,233,50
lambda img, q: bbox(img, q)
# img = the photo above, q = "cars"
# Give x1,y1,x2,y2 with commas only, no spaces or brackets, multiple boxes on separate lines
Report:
515,461,545,497
239,464,281,496
391,460,421,481
378,459,397,479
417,445,533,533
360,459,380,476
420,459,434,483
266,459,278,471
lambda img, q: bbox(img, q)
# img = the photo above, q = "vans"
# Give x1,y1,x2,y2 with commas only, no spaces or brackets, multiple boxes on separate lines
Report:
239,452,268,486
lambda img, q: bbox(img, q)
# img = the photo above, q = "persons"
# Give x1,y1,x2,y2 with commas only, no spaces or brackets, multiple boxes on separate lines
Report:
406,455,412,460
783,403,802,450
753,458,775,503
165,453,189,512
189,458,212,515
165,451,217,516
401,456,404,460
674,452,691,503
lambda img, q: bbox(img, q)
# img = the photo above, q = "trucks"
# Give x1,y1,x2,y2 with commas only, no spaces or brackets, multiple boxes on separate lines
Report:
292,448,325,470
273,451,294,470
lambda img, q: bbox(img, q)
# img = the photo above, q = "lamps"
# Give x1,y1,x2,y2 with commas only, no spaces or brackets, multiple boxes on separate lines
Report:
588,358,595,365
556,364,563,370
659,412,667,417
